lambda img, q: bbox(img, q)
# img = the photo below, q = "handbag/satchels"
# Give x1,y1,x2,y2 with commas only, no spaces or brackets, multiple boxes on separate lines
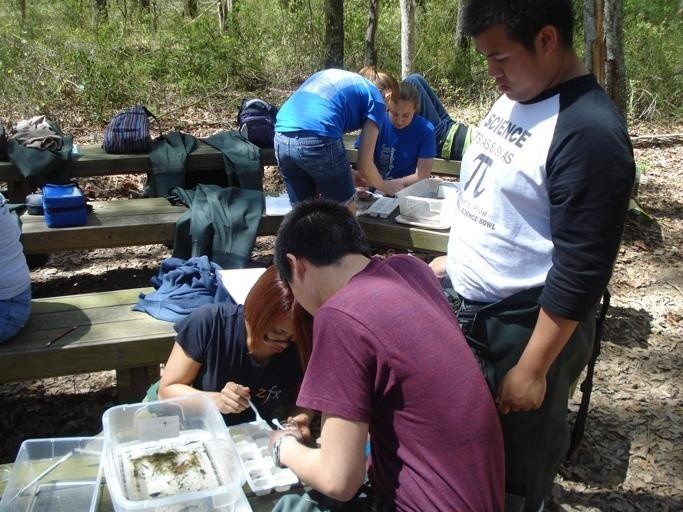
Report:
26,192,47,215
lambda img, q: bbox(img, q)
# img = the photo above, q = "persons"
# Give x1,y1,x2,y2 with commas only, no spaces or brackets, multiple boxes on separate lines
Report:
428,0,636,512
351,83,437,196
271,199,510,512
403,71,477,160
1,191,32,346
274,65,404,216
138,263,315,424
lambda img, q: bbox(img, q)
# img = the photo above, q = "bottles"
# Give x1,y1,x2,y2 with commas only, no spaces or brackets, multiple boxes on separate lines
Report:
276,418,300,432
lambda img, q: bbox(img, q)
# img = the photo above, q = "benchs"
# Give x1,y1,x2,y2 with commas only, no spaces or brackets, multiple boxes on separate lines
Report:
0,424,375,512
1,278,254,407
3,146,460,189
1,189,460,253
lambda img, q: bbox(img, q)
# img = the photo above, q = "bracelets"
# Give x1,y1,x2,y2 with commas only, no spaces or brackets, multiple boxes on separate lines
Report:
273,433,296,468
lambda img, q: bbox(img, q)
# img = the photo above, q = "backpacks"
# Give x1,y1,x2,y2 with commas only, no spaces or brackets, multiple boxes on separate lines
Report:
42,179,90,228
102,105,152,152
236,97,277,147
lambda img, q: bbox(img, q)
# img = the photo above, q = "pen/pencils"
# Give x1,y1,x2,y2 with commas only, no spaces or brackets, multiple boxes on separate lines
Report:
355,188,375,195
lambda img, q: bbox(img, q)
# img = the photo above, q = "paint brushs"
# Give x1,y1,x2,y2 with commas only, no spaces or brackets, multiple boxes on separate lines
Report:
45,326,77,347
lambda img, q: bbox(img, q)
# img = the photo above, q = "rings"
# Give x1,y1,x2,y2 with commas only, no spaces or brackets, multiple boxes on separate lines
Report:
234,383,238,392
